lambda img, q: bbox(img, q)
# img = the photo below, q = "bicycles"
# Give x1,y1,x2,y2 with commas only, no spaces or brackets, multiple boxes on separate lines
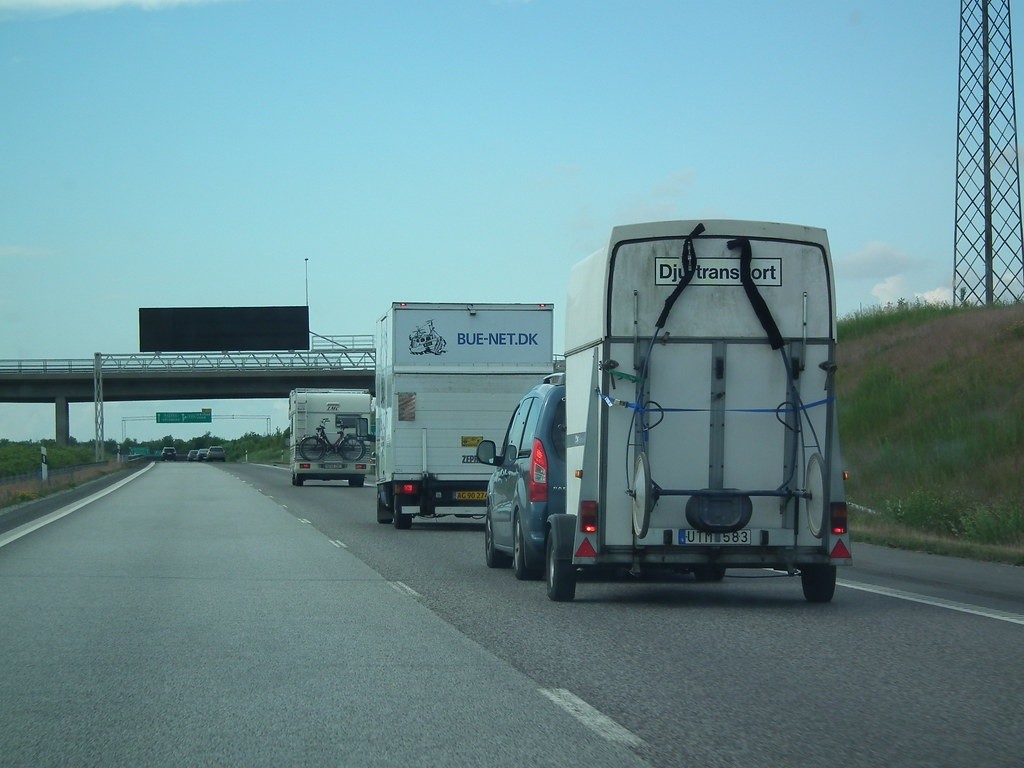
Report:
299,418,372,462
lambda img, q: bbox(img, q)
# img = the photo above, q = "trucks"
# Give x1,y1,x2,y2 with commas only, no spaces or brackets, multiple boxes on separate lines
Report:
375,302,554,530
288,388,372,487
548,220,850,601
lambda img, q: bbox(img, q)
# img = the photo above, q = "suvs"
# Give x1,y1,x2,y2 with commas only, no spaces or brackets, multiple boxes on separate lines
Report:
478,374,567,580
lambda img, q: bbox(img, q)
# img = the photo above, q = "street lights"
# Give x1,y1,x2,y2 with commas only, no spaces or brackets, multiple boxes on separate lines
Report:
305,258,308,306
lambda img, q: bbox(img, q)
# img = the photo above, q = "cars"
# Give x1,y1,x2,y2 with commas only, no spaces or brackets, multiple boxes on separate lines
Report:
161,447,177,461
206,446,226,462
196,449,209,462
187,450,199,461
